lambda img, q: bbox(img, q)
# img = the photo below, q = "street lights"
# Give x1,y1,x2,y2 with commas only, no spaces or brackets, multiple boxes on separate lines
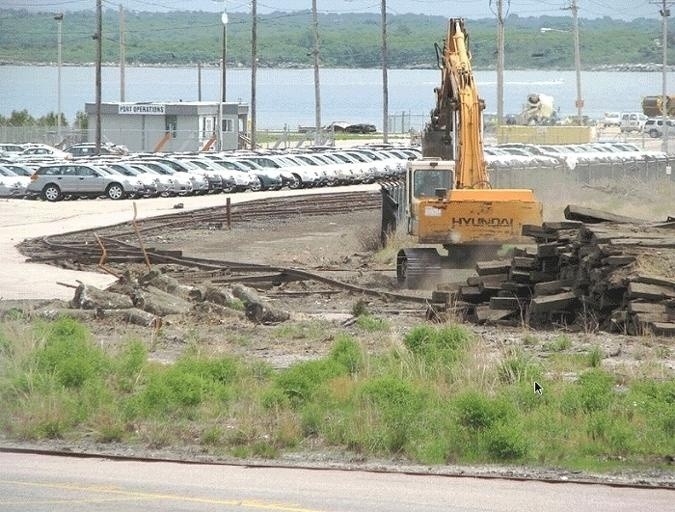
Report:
541,27,581,118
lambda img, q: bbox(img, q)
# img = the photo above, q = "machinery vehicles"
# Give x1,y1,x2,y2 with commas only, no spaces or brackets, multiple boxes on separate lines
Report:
397,16,544,290
642,94,675,118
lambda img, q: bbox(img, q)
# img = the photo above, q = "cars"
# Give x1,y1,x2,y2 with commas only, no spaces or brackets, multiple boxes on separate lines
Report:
604,114,623,128
344,123,377,134
1,141,675,202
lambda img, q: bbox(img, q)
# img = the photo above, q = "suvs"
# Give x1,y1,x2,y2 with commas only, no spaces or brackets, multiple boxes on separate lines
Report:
642,119,675,138
619,114,647,133
